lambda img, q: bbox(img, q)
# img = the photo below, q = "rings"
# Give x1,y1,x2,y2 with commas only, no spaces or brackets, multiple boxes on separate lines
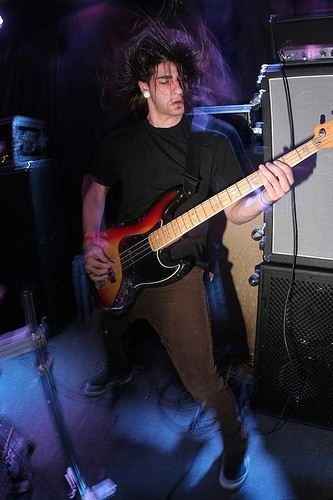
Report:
88,272,94,277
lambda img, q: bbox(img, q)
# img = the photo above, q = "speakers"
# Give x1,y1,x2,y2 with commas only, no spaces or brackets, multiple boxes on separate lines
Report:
250,262,333,432
263,66,333,271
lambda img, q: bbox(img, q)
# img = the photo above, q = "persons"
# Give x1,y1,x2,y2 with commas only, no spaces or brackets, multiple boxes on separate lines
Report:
83,29,294,490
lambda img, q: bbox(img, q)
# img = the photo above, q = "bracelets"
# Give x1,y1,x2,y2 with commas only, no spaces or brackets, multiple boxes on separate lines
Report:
260,188,272,205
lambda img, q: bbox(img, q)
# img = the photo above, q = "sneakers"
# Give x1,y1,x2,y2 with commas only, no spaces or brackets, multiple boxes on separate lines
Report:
84,361,132,396
219,433,247,490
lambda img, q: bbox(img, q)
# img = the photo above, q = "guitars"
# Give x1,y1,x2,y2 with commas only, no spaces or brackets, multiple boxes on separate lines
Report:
85,112,332,318
